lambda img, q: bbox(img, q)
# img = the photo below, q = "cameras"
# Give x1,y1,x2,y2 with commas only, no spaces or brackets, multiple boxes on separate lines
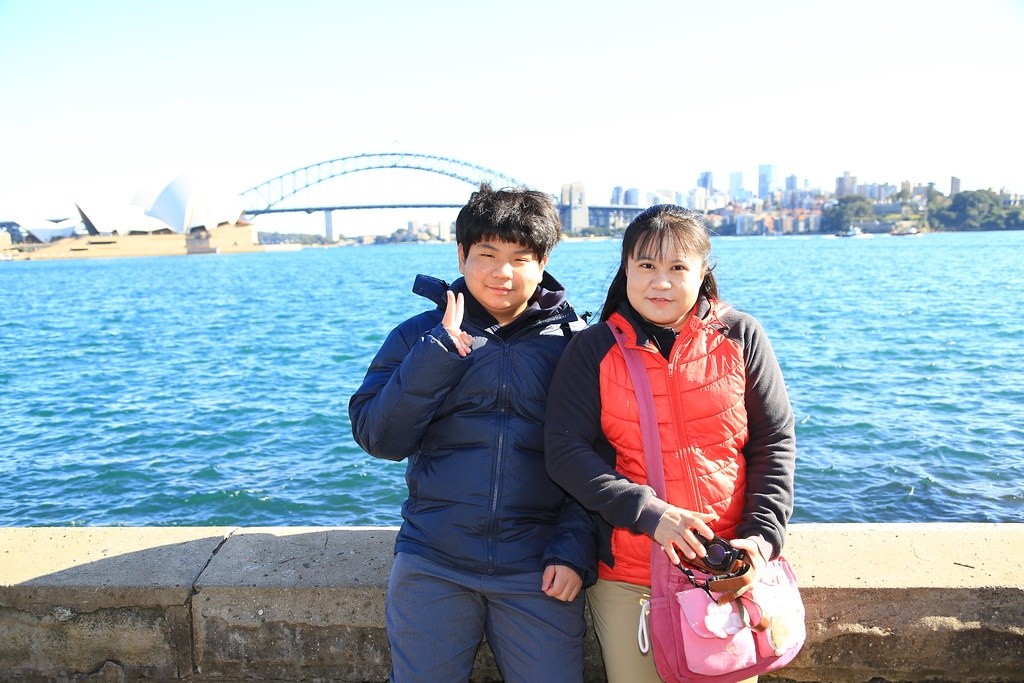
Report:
681,529,740,575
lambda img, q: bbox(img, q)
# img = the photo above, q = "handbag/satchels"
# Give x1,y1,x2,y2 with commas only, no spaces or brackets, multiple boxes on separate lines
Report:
637,538,806,683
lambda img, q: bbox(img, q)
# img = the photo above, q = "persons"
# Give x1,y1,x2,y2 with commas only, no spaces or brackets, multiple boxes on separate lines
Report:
545,204,796,683
349,182,599,683
